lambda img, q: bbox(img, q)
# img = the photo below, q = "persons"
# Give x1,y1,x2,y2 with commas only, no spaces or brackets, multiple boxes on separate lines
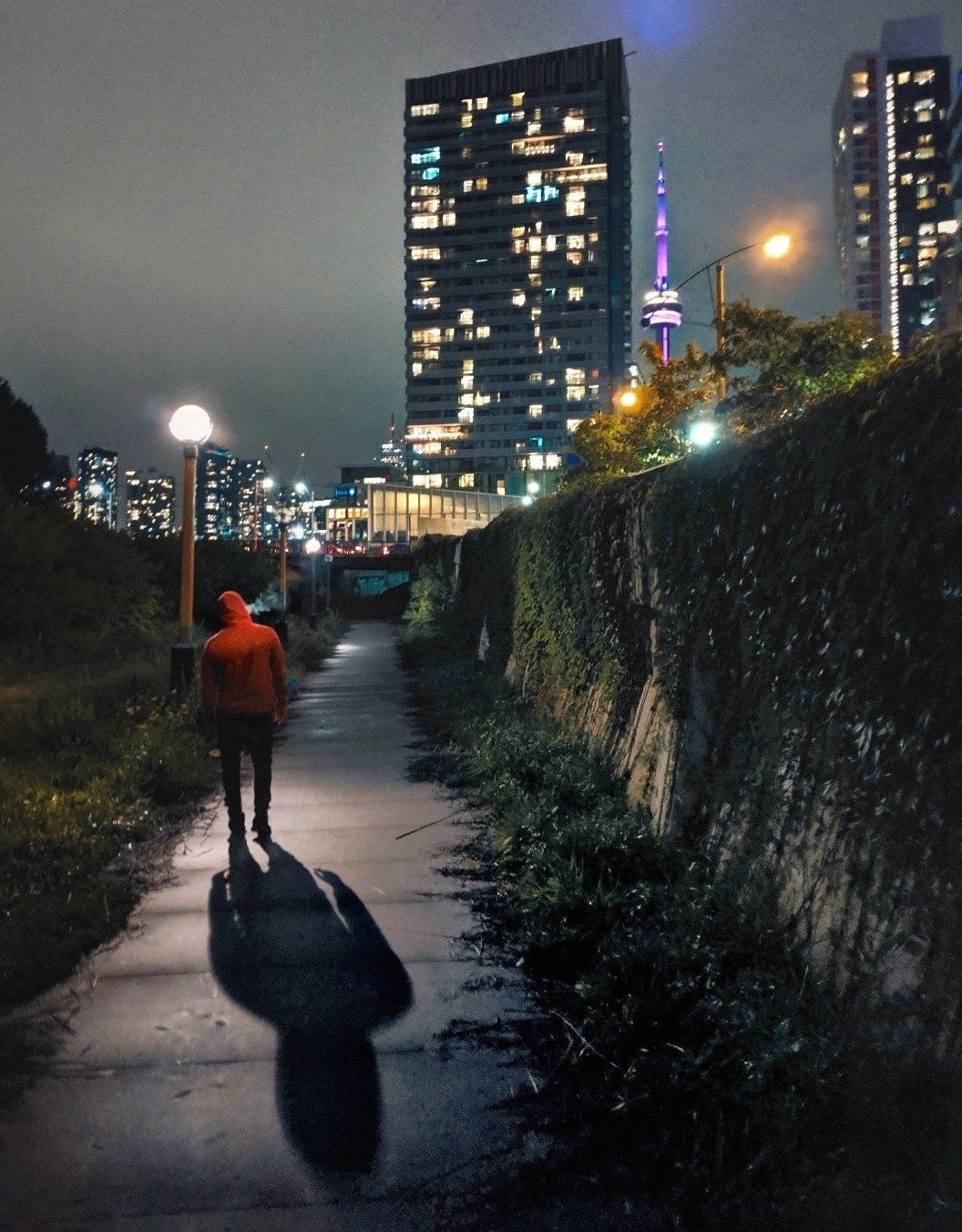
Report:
202,592,287,835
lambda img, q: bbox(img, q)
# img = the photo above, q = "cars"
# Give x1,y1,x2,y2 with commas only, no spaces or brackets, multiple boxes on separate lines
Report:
325,538,411,555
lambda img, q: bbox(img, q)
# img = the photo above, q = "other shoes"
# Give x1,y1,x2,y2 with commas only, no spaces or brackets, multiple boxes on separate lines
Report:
227,822,246,832
252,817,269,826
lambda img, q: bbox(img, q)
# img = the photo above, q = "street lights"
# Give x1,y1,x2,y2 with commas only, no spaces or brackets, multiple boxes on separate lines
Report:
672,232,793,295
305,535,321,630
170,404,214,687
